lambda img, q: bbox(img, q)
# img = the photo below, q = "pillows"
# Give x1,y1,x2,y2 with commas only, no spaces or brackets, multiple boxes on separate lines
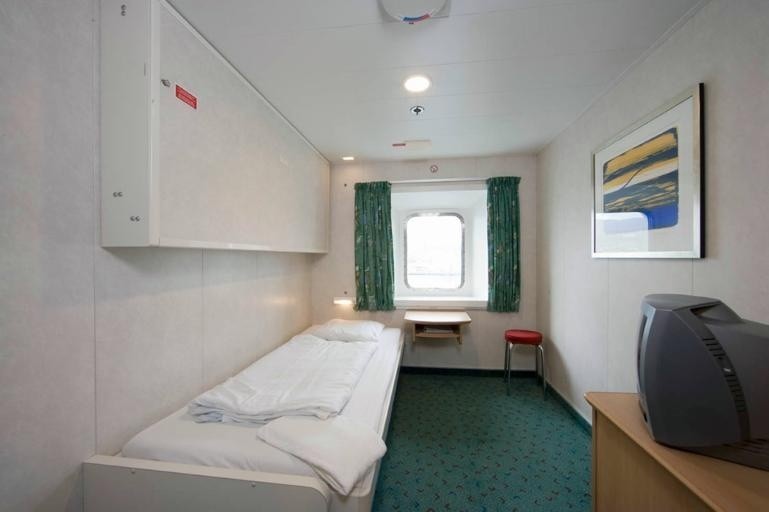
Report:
313,319,385,343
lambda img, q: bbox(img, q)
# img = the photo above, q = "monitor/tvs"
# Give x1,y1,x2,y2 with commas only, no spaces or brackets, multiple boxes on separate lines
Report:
636,293,769,475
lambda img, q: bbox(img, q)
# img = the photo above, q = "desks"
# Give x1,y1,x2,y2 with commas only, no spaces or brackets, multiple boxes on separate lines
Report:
404,309,471,344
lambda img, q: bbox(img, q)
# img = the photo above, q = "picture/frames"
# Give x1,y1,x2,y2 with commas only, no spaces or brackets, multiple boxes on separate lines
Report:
590,82,705,261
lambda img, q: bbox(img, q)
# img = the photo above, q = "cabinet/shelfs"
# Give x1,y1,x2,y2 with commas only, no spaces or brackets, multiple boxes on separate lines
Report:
583,391,765,512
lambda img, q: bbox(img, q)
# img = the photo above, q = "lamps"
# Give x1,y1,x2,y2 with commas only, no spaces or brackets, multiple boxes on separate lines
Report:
381,0,446,25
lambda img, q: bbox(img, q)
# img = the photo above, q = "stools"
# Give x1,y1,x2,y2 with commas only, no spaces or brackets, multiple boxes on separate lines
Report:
504,330,547,402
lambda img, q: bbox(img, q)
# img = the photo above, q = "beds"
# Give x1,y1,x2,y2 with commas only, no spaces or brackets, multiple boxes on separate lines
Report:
82,318,404,512
